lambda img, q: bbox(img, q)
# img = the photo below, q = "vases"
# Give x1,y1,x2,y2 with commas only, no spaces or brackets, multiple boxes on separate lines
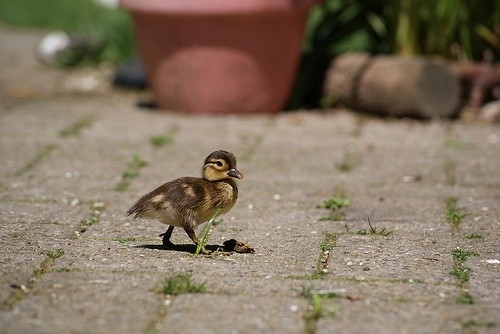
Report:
118,1,330,115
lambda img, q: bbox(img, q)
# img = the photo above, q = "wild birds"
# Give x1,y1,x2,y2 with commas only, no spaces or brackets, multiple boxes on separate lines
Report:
124,150,243,256
